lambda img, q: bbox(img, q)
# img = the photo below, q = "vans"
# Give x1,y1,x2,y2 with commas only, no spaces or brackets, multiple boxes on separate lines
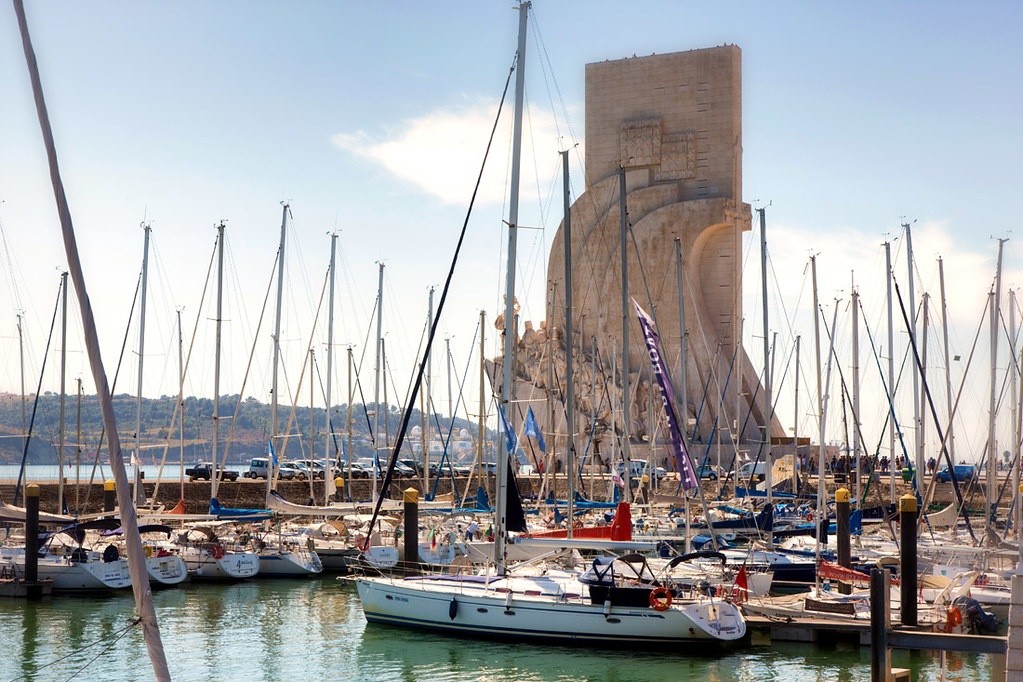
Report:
356,458,399,479
728,461,766,482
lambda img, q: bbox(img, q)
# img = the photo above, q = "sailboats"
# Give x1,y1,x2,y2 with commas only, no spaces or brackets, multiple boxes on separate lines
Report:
0,0,1023,657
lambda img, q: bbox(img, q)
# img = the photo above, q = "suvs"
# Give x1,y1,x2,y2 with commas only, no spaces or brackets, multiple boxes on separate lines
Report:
323,459,362,480
249,458,297,480
399,459,438,478
616,458,672,481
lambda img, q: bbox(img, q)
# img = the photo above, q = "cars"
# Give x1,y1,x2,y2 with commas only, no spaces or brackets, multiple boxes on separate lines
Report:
697,464,720,480
395,460,416,478
298,459,346,479
471,462,497,476
350,463,380,479
184,462,239,480
242,469,250,478
283,462,319,480
436,462,471,477
934,465,978,481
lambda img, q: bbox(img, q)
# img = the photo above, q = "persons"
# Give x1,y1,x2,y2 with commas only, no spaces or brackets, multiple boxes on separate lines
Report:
673,456,677,472
830,454,904,474
334,474,344,502
557,459,561,472
797,454,814,474
927,457,935,475
694,457,698,465
466,521,479,542
605,458,609,473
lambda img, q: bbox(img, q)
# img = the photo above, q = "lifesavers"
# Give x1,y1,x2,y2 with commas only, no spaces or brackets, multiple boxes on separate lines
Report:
573,521,584,530
692,517,699,524
949,607,962,627
806,512,813,522
211,545,226,560
715,586,729,600
356,536,371,552
143,545,153,557
648,586,672,612
738,589,748,602
